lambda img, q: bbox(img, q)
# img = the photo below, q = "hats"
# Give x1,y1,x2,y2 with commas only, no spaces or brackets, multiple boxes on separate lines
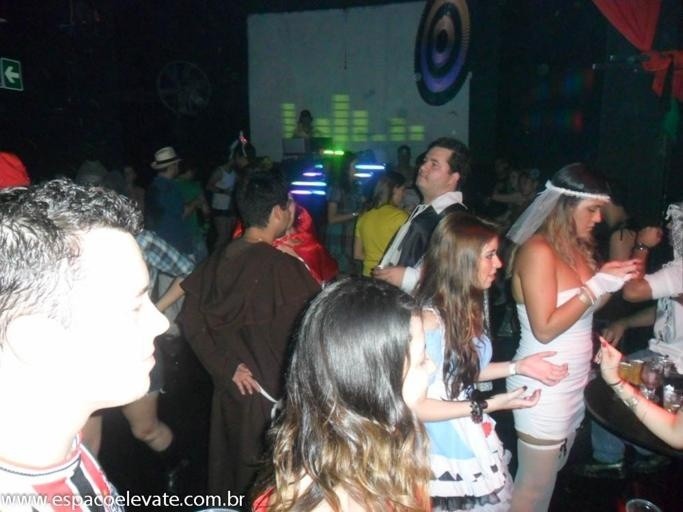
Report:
150,145,181,169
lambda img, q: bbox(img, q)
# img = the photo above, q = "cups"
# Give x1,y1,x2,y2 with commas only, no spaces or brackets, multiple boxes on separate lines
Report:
616,359,682,413
624,498,661,512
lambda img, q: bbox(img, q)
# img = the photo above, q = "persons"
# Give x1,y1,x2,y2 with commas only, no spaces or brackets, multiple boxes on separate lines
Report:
292,108,313,140
